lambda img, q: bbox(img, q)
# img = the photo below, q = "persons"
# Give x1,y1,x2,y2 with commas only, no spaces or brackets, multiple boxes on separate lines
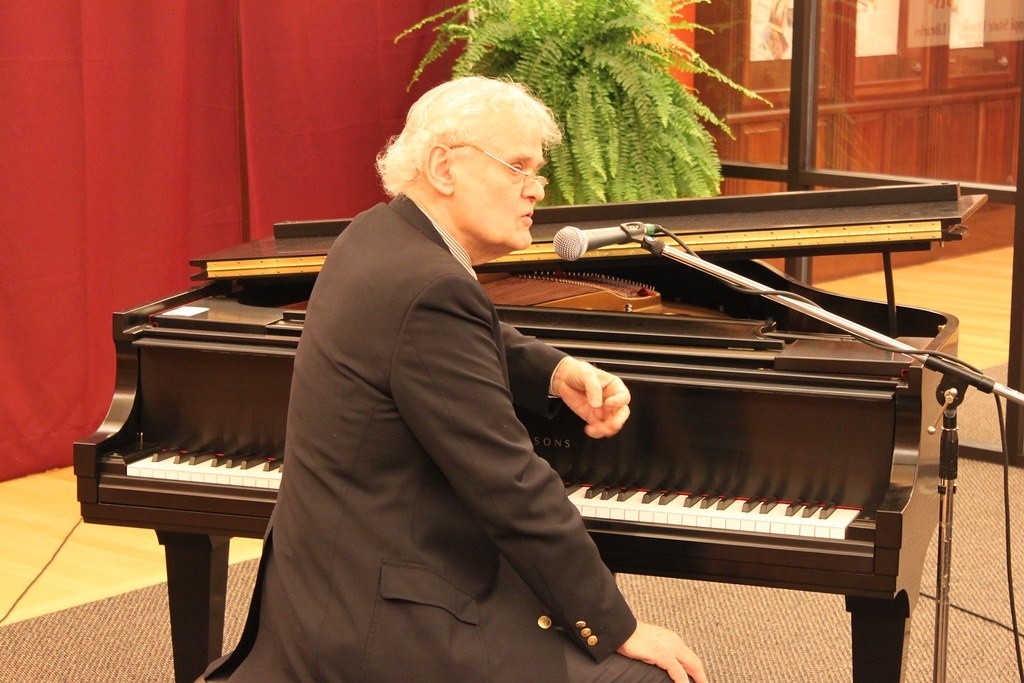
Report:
199,74,710,683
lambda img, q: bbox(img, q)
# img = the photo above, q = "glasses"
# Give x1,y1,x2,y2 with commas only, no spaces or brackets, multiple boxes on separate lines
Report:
449,143,549,188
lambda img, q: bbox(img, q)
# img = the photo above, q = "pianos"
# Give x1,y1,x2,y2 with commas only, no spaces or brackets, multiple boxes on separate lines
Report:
73,180,990,683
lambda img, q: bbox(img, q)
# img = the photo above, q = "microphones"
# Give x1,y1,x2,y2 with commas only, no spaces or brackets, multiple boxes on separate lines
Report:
553,222,663,261
